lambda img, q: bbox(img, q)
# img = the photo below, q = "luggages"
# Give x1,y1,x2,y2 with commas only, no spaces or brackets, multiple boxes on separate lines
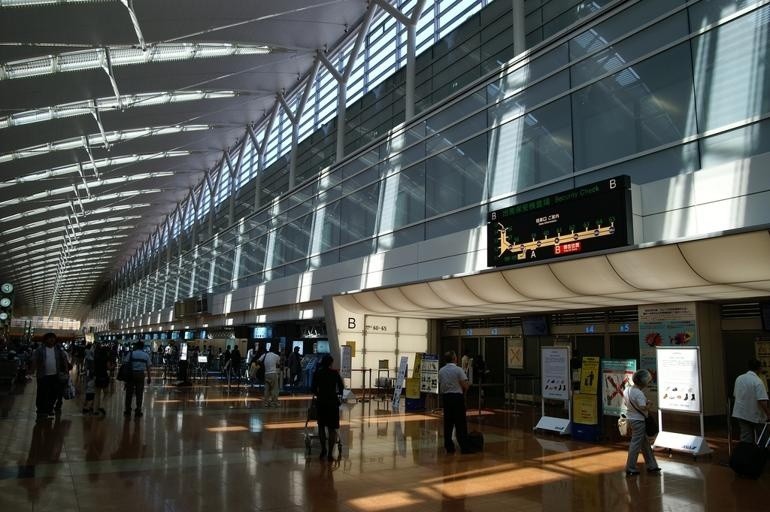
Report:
730,442,764,480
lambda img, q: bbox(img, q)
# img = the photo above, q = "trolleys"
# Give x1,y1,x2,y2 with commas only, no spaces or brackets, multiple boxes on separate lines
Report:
304,392,343,454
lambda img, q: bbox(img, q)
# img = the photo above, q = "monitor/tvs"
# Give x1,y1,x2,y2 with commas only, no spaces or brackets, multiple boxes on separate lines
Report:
98,329,207,341
253,327,268,339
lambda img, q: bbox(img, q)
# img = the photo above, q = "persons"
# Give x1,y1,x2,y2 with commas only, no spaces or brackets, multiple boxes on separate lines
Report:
159,339,212,381
33,333,72,421
309,354,345,462
621,368,662,475
75,340,134,415
246,343,302,386
437,350,470,454
217,344,241,375
731,363,770,442
259,346,281,406
124,340,152,416
3,340,39,384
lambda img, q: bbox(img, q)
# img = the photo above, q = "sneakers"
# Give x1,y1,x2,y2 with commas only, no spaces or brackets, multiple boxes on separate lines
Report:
647,468,661,472
626,471,640,474
124,412,143,417
265,402,280,407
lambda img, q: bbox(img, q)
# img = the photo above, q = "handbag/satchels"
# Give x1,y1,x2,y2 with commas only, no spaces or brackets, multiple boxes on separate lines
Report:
117,364,131,382
618,415,631,437
258,362,264,377
309,398,319,419
468,431,484,452
645,416,658,436
65,384,76,399
